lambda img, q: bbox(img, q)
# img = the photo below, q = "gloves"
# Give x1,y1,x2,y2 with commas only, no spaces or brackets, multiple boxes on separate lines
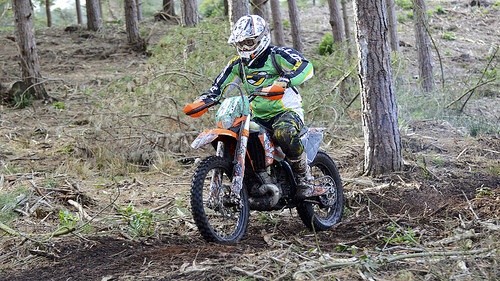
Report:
193,96,214,106
273,78,291,90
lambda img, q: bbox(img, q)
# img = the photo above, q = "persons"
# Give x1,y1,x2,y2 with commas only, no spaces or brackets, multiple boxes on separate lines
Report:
193,14,315,199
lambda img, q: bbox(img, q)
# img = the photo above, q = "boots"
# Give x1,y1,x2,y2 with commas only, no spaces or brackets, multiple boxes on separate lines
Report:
288,152,315,199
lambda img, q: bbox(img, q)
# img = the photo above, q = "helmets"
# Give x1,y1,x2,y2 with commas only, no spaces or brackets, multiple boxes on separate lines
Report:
227,14,272,60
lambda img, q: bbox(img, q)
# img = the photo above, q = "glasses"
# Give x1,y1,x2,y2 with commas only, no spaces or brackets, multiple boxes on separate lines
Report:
236,23,268,50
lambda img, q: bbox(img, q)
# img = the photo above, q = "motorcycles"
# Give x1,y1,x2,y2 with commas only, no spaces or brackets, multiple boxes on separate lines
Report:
190,92,345,245
